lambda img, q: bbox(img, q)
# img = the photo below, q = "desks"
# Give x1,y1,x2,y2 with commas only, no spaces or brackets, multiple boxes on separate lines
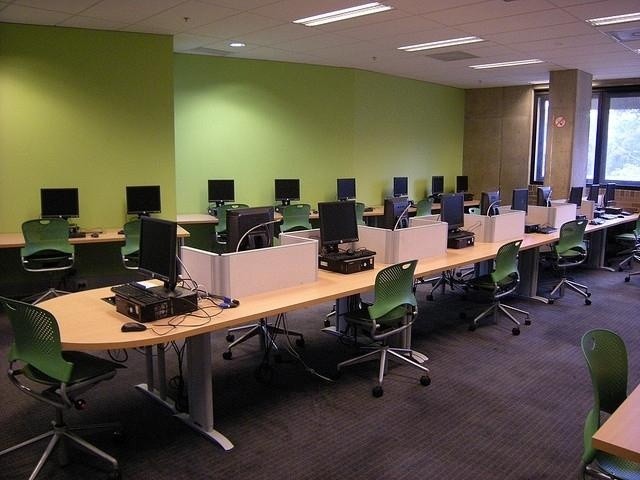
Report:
176,198,504,226
32,211,640,453
0,222,191,250
591,382,639,467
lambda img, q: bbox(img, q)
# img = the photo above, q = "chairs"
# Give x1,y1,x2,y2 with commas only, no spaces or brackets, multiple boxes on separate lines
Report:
355,202,366,226
272,203,313,233
119,217,141,272
413,271,466,301
578,327,630,480
539,219,592,307
18,215,76,307
2,296,128,480
323,303,336,327
456,200,481,291
214,202,251,246
221,235,307,364
335,259,432,397
614,217,640,273
416,197,436,218
470,238,532,337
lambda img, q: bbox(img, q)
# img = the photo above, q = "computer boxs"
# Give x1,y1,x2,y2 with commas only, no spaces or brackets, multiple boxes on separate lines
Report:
447,235,475,249
593,211,604,218
113,285,199,324
606,208,625,213
319,257,374,274
525,225,540,234
576,216,587,222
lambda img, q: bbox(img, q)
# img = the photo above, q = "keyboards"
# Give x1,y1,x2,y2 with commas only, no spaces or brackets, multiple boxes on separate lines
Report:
601,214,618,220
118,229,124,234
448,231,475,240
324,249,377,262
68,232,86,238
540,227,558,234
110,281,169,308
589,217,606,225
620,210,632,215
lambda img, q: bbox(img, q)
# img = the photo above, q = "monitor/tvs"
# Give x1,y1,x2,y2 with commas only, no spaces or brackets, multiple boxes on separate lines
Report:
393,177,408,197
587,184,600,204
511,188,529,215
126,185,162,219
207,179,235,208
318,199,359,254
384,196,409,228
440,194,465,231
337,178,356,202
40,188,80,220
274,178,300,206
137,215,182,298
603,183,615,207
432,175,444,195
480,191,501,216
536,186,552,207
456,175,469,193
225,205,275,254
570,186,584,209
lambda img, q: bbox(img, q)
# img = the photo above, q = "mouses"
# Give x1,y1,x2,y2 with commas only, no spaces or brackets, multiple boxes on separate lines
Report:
91,233,98,238
314,209,316,214
347,249,356,255
121,322,147,333
618,215,625,218
